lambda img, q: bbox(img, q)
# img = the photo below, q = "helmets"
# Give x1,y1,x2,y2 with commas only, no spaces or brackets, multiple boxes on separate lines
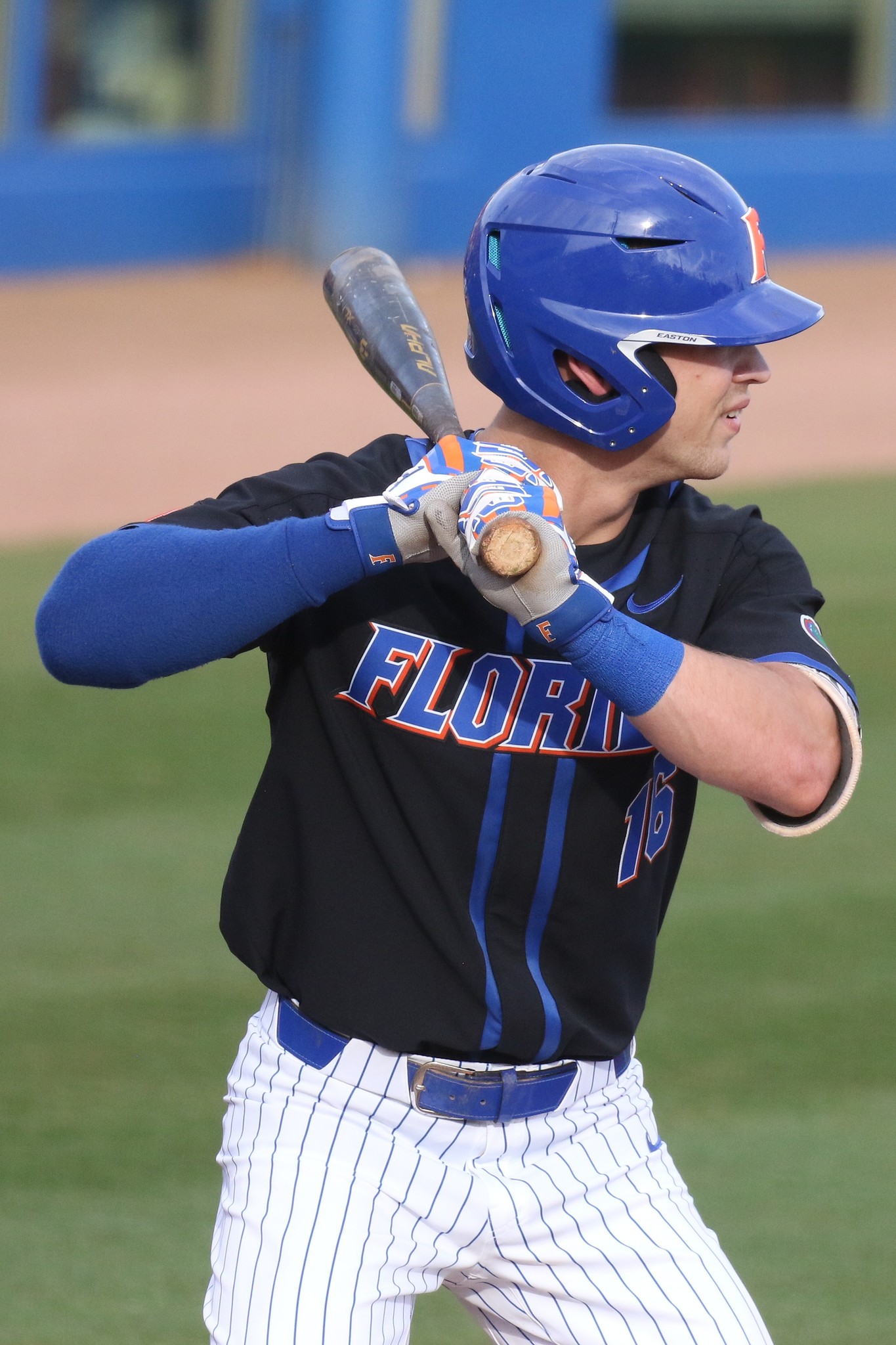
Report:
462,143,825,451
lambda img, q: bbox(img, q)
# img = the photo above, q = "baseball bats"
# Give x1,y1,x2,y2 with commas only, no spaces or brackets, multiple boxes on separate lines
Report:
323,246,542,578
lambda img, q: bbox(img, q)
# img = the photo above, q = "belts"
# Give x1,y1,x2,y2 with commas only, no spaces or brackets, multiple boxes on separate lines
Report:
276,1000,631,1123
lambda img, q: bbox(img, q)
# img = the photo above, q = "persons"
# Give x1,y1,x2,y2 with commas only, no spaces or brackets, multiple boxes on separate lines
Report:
36,144,865,1345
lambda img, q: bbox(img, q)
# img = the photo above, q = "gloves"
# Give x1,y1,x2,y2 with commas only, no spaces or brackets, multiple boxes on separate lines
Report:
424,453,616,651
324,435,563,576
